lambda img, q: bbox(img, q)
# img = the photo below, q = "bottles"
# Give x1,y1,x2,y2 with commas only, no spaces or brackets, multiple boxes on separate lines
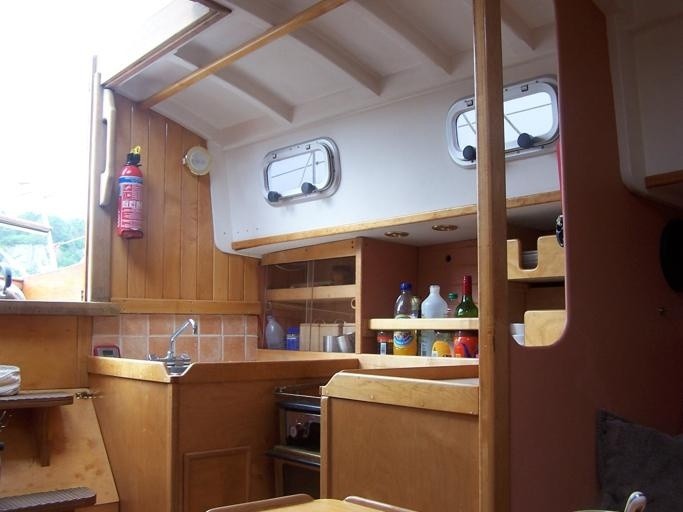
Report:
394,275,479,357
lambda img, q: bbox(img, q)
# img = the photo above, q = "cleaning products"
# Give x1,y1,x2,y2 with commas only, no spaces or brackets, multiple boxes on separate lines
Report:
421,284,448,356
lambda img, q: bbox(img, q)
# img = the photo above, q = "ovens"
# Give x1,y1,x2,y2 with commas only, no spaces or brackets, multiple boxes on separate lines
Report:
267,401,319,501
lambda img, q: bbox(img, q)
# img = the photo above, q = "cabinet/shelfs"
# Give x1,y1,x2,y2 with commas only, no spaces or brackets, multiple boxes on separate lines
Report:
259,236,417,355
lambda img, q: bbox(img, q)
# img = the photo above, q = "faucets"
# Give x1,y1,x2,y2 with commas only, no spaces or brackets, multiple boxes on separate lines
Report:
165,318,198,362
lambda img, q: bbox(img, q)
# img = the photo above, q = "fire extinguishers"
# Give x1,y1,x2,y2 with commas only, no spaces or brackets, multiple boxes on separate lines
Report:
116,146,145,239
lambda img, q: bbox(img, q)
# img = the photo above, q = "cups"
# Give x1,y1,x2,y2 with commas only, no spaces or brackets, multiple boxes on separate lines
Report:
322,333,357,354
